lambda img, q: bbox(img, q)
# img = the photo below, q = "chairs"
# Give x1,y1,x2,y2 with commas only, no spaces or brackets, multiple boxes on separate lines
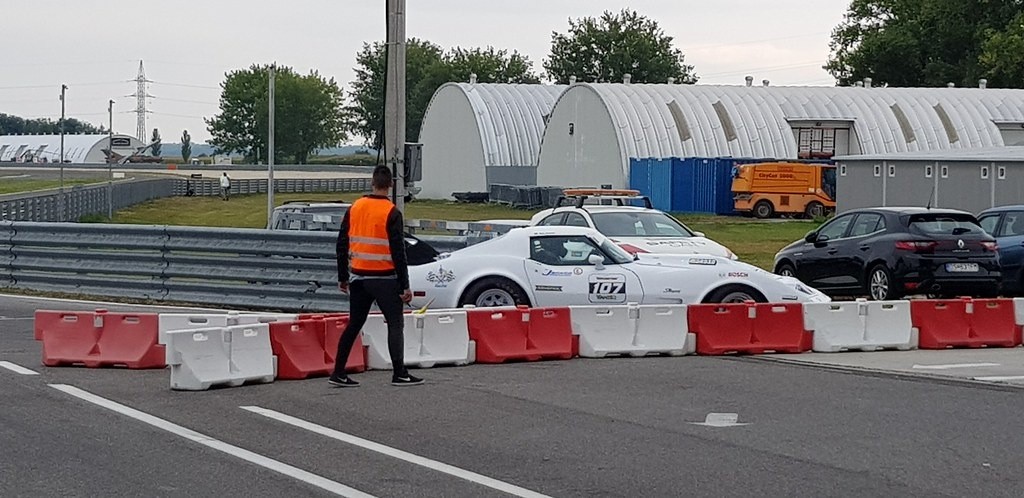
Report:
1012,212,1024,235
865,221,882,233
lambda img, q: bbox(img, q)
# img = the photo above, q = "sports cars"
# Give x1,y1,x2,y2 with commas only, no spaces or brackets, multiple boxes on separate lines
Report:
406,226,833,314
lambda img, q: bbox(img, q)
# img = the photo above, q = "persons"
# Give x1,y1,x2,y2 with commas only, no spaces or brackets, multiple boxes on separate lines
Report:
328,169,426,387
537,238,599,265
220,172,233,200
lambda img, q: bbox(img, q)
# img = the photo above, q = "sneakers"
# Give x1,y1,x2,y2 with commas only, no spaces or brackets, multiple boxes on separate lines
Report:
328,371,360,387
390,373,426,385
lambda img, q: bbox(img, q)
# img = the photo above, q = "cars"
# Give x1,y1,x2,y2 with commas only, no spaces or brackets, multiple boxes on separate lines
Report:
975,206,1024,297
531,184,738,264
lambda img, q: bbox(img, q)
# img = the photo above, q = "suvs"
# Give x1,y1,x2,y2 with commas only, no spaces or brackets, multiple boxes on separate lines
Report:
772,207,1003,302
263,199,354,232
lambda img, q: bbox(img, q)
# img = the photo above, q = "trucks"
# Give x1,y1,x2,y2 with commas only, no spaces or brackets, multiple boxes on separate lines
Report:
730,161,837,221
